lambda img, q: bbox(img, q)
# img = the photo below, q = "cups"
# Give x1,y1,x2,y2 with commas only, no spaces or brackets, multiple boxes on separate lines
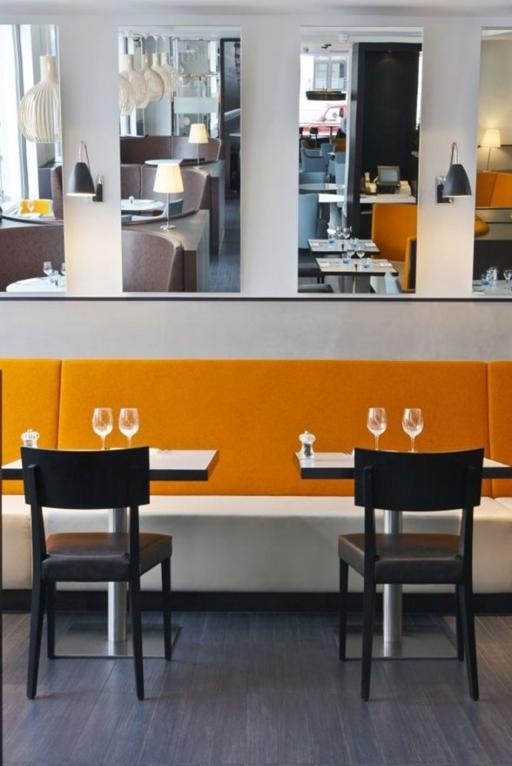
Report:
17,198,54,219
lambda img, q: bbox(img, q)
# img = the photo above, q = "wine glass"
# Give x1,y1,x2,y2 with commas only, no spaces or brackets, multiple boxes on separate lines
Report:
93,407,113,449
347,237,365,271
481,267,511,294
119,406,139,449
366,407,387,451
42,259,65,288
327,225,350,248
402,407,424,454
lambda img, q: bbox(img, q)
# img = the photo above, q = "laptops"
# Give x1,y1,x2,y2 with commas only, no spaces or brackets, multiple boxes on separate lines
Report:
379,165,401,189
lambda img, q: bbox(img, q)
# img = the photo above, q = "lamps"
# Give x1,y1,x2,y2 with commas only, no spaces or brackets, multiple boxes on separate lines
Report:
19,32,177,143
187,123,209,170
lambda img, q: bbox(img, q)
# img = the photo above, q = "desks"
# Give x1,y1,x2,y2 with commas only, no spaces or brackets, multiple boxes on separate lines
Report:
293,452,511,660
0,450,219,659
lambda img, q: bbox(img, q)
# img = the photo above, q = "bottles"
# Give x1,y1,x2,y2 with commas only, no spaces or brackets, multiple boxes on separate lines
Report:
297,430,316,459
21,429,41,448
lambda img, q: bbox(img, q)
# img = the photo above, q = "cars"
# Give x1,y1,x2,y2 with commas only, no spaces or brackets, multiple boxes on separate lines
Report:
299,102,347,136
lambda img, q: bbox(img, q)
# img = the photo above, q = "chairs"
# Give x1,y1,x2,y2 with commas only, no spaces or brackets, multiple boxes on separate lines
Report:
338,447,484,701
20,447,171,700
